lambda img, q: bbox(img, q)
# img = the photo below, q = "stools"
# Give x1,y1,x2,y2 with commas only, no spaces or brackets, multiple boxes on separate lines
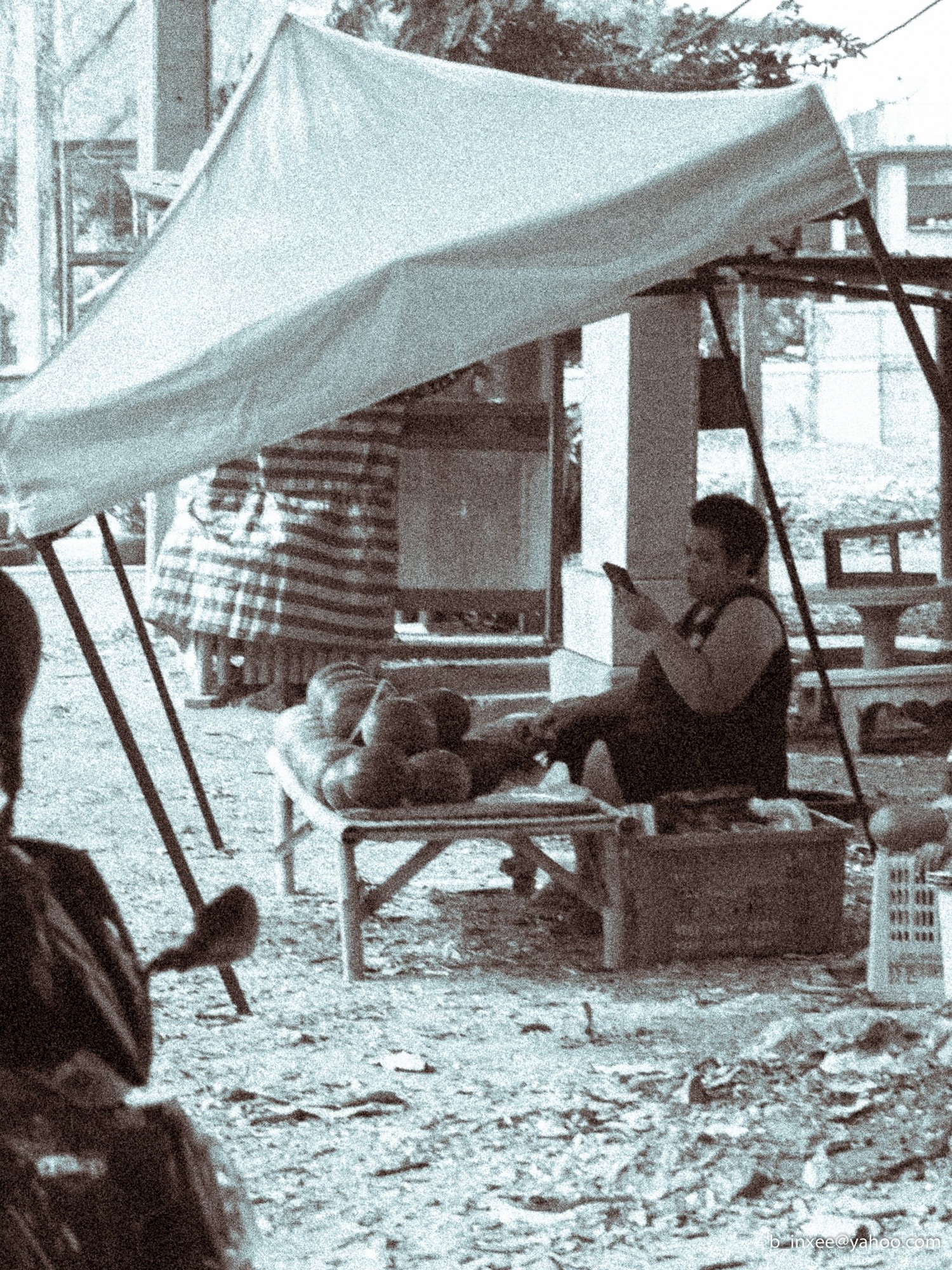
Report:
823,519,936,588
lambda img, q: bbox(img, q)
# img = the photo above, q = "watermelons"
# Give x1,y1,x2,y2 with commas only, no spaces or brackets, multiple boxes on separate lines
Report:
272,661,508,810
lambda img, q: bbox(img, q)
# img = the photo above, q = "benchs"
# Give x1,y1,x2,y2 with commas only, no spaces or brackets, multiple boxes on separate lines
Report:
788,634,952,736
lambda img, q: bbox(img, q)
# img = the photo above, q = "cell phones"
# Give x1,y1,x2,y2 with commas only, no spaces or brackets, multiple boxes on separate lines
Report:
602,562,637,597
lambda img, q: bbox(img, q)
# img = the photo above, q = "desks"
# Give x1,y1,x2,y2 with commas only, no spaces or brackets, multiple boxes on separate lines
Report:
266,743,642,980
792,579,952,669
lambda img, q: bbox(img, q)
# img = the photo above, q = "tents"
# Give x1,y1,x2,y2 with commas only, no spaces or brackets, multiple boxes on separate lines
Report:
0,12,952,1014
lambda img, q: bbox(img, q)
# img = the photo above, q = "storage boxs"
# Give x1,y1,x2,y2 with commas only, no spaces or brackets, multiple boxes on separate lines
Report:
868,849,946,1006
573,797,854,967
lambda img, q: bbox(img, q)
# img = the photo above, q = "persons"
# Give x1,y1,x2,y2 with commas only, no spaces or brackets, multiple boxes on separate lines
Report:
501,493,790,936
